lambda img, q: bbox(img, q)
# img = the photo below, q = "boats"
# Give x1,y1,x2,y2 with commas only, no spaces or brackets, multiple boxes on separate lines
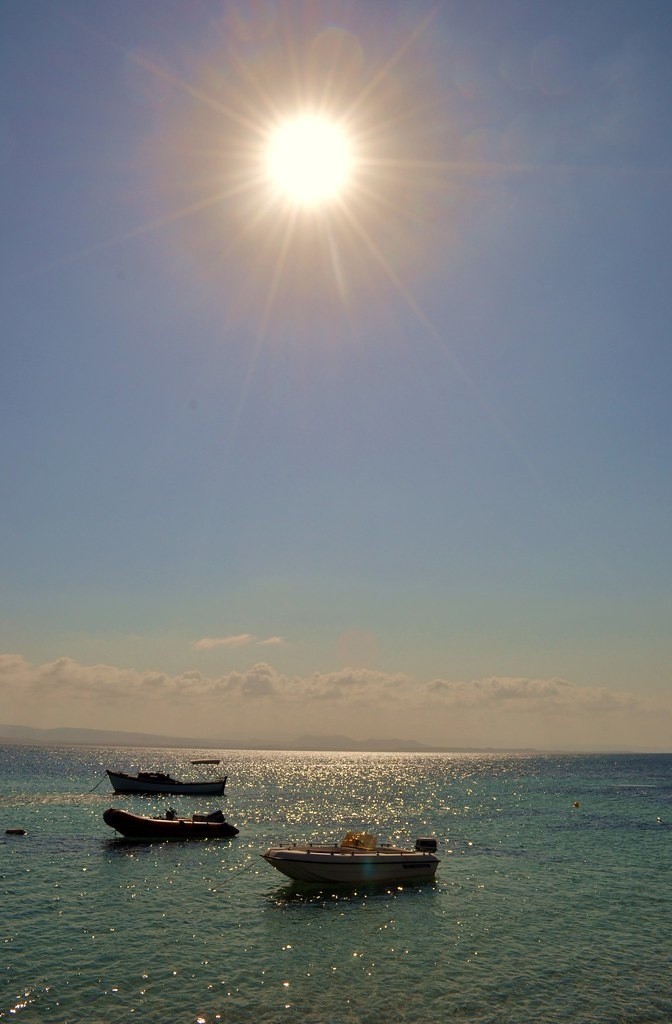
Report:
260,831,441,890
103,807,240,839
106,759,228,797
